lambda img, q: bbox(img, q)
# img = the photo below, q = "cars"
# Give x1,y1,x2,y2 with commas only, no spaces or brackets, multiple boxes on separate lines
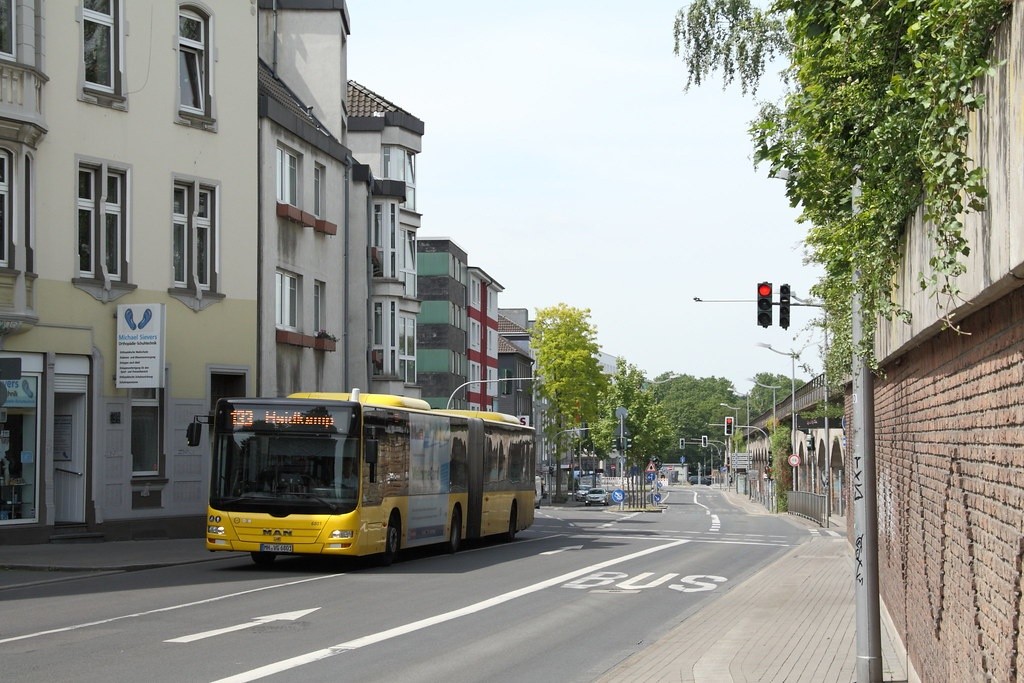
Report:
575,484,592,502
690,476,711,486
584,488,609,506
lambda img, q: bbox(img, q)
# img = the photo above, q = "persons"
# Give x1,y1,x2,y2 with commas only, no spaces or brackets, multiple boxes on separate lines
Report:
329,460,358,487
728,475,732,486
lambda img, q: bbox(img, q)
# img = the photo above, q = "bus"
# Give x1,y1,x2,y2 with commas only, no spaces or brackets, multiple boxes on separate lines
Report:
185,387,536,568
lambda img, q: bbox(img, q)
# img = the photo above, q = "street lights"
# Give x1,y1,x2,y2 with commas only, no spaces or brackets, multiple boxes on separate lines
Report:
726,387,752,500
753,341,798,492
718,402,741,494
746,378,782,436
447,376,541,410
549,427,592,506
771,289,832,517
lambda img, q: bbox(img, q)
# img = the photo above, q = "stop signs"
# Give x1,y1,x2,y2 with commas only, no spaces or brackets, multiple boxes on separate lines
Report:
610,465,615,470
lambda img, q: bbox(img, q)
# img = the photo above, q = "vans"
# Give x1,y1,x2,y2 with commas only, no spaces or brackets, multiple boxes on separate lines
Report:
577,475,601,488
535,476,542,510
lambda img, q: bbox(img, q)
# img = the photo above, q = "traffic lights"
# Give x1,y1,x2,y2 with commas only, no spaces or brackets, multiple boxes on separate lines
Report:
757,281,773,329
611,437,621,451
679,438,685,449
779,284,790,331
701,436,708,448
725,416,734,435
623,437,632,452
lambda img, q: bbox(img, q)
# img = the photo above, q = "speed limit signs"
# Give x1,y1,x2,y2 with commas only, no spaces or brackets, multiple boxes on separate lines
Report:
788,455,801,467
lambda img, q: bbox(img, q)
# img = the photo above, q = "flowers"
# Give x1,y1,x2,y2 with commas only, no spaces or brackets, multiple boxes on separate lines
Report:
315,329,336,340
